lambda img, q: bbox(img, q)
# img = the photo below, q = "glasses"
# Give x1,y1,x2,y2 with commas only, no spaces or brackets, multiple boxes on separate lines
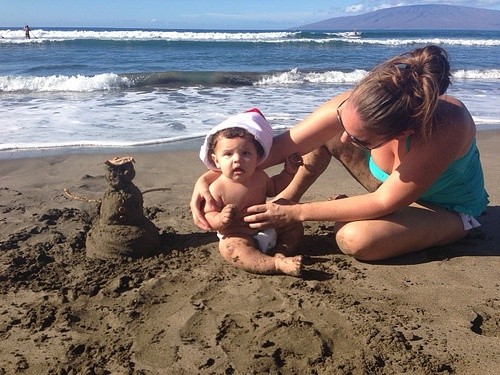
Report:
337,92,403,154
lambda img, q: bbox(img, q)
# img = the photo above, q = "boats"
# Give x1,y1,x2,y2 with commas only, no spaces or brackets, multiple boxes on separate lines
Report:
345,36,361,38
1,38,47,43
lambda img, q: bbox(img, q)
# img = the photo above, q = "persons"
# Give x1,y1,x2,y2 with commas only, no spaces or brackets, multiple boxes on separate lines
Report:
198,108,306,278
23,25,31,39
189,44,491,263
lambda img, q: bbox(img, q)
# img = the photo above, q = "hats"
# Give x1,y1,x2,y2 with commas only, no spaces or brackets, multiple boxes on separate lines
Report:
200,108,275,171
